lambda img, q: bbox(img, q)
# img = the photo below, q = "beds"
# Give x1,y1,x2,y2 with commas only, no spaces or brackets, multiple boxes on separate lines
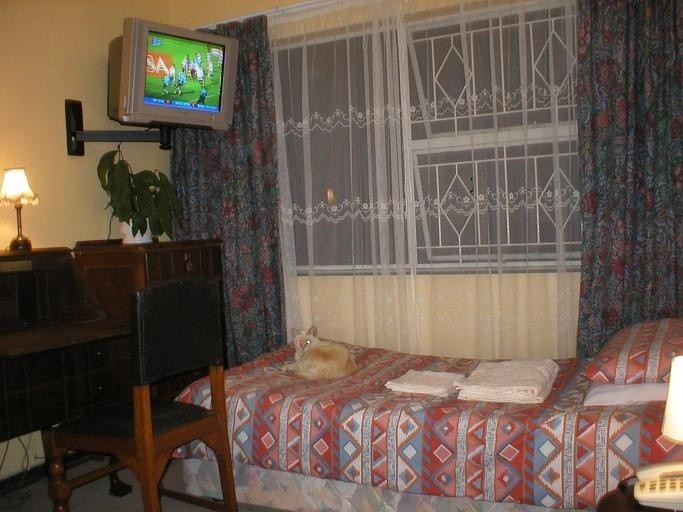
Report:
159,316,683,512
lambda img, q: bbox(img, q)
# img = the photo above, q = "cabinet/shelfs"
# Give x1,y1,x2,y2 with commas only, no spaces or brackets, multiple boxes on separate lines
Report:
75,236,228,404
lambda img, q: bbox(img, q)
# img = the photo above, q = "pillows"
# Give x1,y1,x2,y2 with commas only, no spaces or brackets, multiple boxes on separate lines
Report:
580,317,682,407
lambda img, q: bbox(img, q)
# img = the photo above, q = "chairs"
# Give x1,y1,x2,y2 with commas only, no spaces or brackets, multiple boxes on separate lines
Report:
42,280,238,511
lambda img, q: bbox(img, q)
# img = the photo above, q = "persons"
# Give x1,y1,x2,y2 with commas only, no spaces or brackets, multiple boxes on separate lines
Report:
153,47,224,106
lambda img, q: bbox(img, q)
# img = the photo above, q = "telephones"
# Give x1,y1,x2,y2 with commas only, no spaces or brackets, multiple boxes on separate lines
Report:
633,461,683,511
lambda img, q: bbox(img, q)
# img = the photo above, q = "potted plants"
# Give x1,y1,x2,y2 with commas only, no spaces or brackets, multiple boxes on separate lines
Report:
95,143,185,245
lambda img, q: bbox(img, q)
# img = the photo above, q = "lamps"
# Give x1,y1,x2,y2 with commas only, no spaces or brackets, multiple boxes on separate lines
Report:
0,168,40,251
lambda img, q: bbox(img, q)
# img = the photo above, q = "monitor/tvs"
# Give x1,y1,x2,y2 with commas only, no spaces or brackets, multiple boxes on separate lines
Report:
108,17,239,131
